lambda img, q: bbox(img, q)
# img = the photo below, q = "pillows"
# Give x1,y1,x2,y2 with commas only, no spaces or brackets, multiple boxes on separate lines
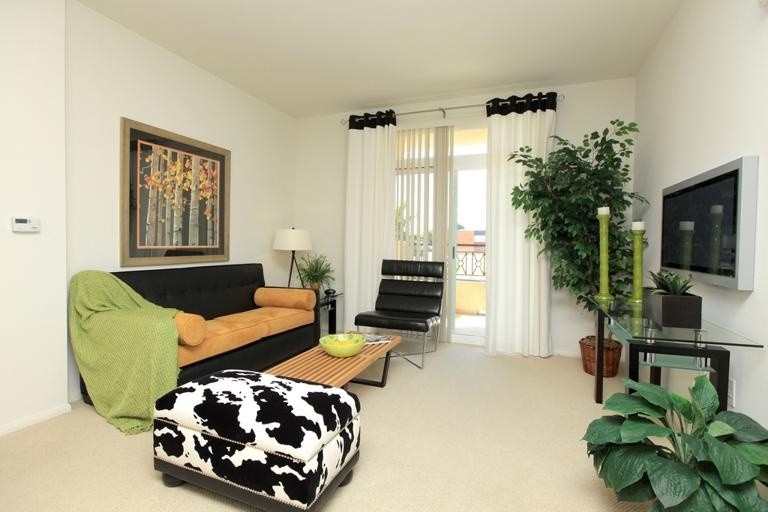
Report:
174,312,207,346
253,287,316,311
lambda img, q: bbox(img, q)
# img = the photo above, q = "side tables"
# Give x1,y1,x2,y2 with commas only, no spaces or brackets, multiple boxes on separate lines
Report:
320,298,337,335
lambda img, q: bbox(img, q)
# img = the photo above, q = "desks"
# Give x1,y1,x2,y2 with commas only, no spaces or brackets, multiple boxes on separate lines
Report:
588,296,764,421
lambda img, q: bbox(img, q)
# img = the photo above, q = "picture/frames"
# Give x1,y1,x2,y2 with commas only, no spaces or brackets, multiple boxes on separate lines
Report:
120,116,231,267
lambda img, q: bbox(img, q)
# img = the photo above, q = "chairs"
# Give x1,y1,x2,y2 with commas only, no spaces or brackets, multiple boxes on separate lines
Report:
355,260,444,371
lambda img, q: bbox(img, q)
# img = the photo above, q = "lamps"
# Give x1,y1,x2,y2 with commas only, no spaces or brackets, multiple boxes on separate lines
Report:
273,225,313,289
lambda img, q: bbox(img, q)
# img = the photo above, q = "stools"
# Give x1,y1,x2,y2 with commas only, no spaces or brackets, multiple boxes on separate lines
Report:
153,368,362,512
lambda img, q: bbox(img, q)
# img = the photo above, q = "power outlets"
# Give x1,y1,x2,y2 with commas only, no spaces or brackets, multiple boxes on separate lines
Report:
728,377,736,408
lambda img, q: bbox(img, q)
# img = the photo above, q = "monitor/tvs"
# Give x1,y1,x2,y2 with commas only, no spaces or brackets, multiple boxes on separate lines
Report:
661,156,758,292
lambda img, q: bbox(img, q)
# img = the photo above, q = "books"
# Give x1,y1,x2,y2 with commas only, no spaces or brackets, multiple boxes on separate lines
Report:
363,332,391,344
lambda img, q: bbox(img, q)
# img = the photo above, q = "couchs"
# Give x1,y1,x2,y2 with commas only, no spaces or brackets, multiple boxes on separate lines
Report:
67,263,320,421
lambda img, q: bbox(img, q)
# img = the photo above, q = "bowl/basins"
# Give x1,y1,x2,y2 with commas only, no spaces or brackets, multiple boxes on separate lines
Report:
319,333,366,358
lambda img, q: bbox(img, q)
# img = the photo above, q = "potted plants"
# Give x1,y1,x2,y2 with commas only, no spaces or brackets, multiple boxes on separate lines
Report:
295,252,335,290
506,119,651,378
643,271,702,331
